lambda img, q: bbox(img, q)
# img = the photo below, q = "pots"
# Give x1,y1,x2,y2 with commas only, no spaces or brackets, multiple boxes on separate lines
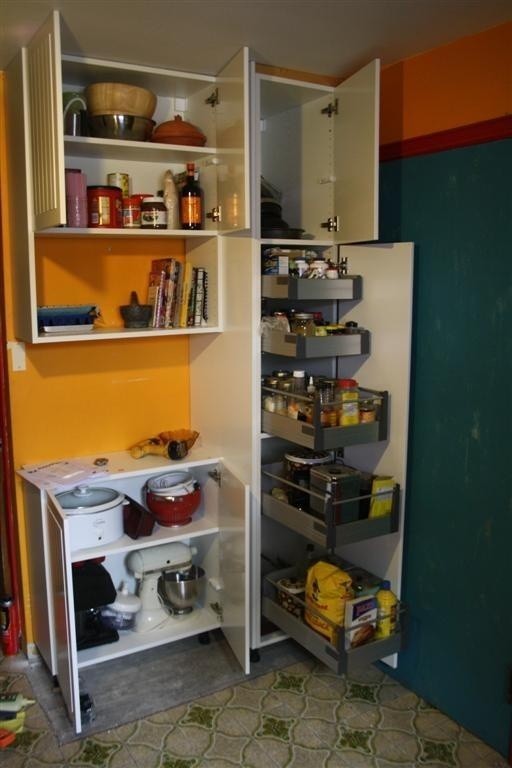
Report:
146,471,200,528
54,482,130,552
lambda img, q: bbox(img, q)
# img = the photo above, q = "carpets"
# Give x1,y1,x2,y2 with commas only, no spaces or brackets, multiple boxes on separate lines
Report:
25,636,307,746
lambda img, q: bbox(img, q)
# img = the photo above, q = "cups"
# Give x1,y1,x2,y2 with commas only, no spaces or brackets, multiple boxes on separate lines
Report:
65,172,88,227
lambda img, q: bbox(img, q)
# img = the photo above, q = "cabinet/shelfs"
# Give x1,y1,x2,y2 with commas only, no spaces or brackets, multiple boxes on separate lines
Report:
21,440,253,735
4,9,253,345
188,241,414,653
254,56,382,245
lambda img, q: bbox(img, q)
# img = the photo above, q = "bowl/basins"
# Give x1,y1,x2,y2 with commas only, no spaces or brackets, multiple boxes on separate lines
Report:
87,113,156,142
119,304,153,328
159,429,200,452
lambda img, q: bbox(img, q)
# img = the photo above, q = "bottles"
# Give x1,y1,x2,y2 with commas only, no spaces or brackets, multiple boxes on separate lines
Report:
374,580,398,641
262,368,376,428
0,595,21,657
292,314,315,338
293,256,339,280
180,163,202,230
